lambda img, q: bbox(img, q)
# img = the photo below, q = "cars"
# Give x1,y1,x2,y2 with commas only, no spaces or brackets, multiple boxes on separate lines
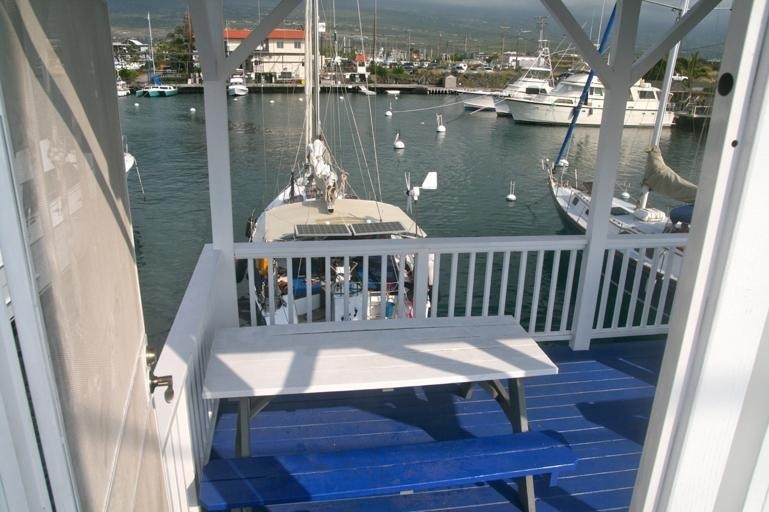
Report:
372,60,509,74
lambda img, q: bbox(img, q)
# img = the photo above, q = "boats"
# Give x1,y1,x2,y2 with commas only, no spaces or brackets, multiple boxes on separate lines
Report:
542,0,693,316
247,0,438,327
116,81,178,97
228,74,249,96
465,0,676,129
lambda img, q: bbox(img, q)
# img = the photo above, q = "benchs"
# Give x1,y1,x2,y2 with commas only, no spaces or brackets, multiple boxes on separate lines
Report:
195,430,578,511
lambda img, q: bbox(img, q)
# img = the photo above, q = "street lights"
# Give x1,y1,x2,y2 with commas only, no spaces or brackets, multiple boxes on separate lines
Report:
146,10,156,85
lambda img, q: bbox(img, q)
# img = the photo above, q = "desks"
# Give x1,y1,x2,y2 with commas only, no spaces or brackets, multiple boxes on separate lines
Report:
200,315,559,511
